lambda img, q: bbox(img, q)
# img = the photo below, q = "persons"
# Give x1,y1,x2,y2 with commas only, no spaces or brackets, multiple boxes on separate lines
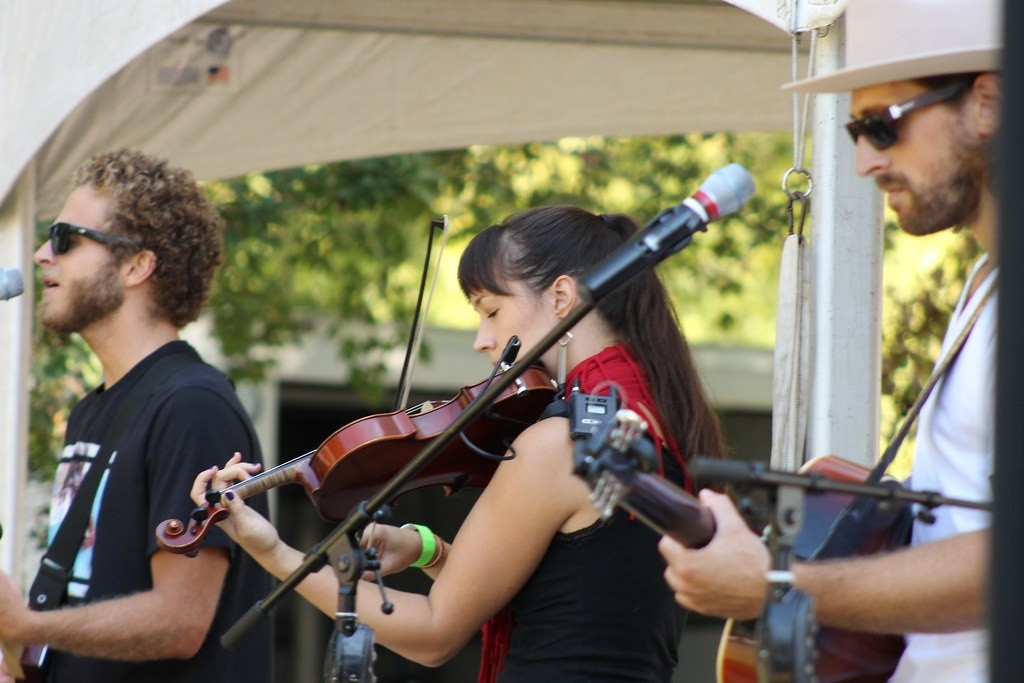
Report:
0,146,270,683
659,0,1002,683
189,207,725,683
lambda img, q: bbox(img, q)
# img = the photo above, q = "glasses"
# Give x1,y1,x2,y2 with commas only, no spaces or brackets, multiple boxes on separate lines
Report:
845,74,982,150
47,222,144,255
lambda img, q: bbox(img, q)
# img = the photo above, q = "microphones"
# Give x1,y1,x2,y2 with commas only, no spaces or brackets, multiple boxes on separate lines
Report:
0,268,24,300
578,163,755,305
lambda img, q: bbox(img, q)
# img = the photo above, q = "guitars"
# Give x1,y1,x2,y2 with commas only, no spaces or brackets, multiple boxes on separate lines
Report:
569,407,911,682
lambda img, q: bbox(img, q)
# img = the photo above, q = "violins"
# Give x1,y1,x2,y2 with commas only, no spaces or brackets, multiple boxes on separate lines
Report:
153,363,561,559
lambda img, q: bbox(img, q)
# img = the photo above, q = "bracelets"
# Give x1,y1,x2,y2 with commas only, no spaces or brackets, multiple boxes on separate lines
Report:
400,524,444,568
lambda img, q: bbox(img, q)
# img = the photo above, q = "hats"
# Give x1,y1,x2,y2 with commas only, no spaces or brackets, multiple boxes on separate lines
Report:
780,0,1006,94
